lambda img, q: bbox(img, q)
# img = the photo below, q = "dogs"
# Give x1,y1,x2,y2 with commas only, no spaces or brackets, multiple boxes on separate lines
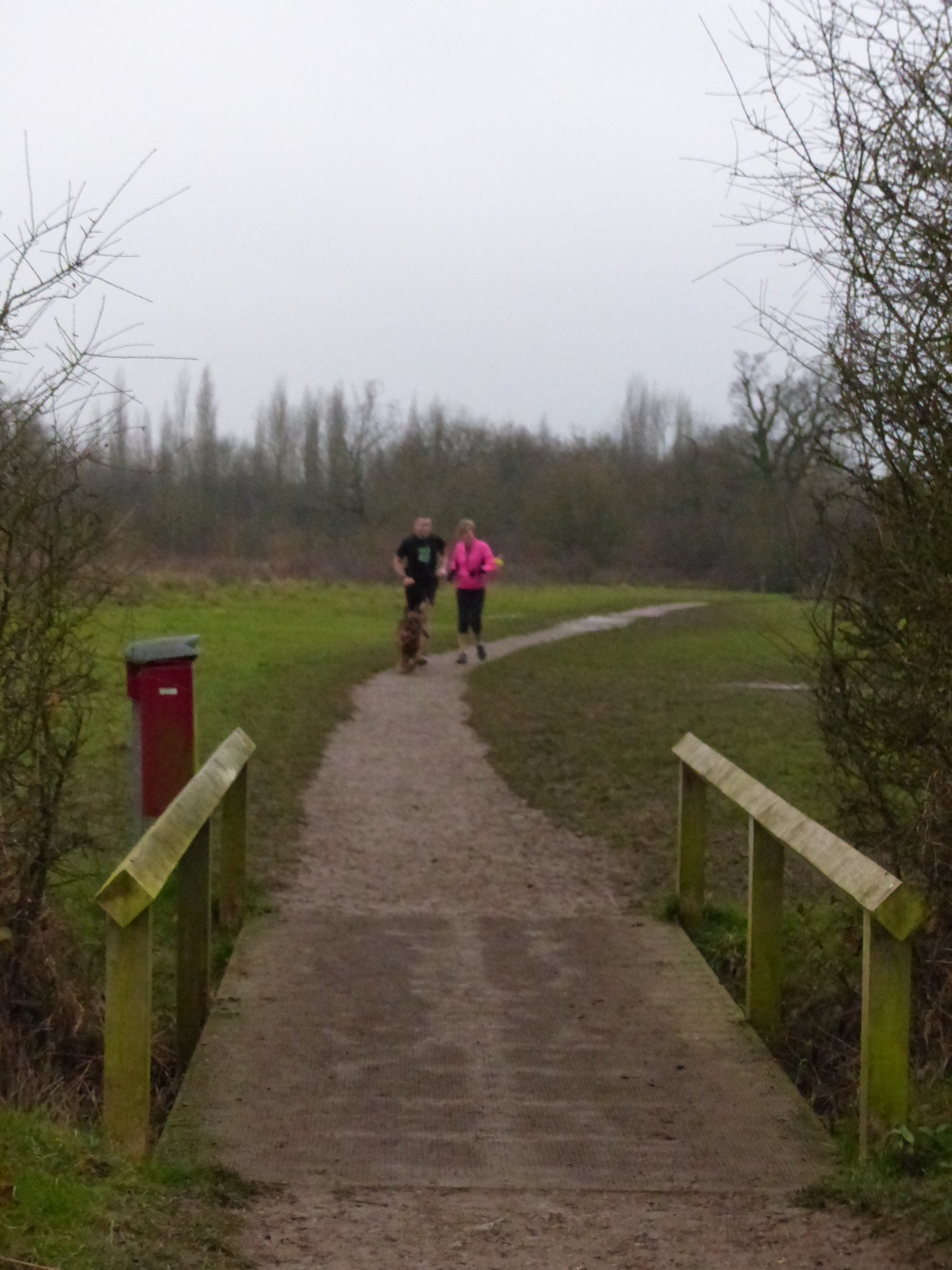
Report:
399,612,430,675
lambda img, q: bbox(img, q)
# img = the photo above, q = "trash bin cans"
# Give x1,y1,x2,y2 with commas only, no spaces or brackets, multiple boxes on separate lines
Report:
125,632,198,816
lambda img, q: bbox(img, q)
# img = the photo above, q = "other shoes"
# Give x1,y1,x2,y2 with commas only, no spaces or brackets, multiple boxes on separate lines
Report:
477,645,487,661
456,653,467,663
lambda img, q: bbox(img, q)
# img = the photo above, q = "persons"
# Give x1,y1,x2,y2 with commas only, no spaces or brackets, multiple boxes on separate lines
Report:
392,514,449,666
447,519,497,664
